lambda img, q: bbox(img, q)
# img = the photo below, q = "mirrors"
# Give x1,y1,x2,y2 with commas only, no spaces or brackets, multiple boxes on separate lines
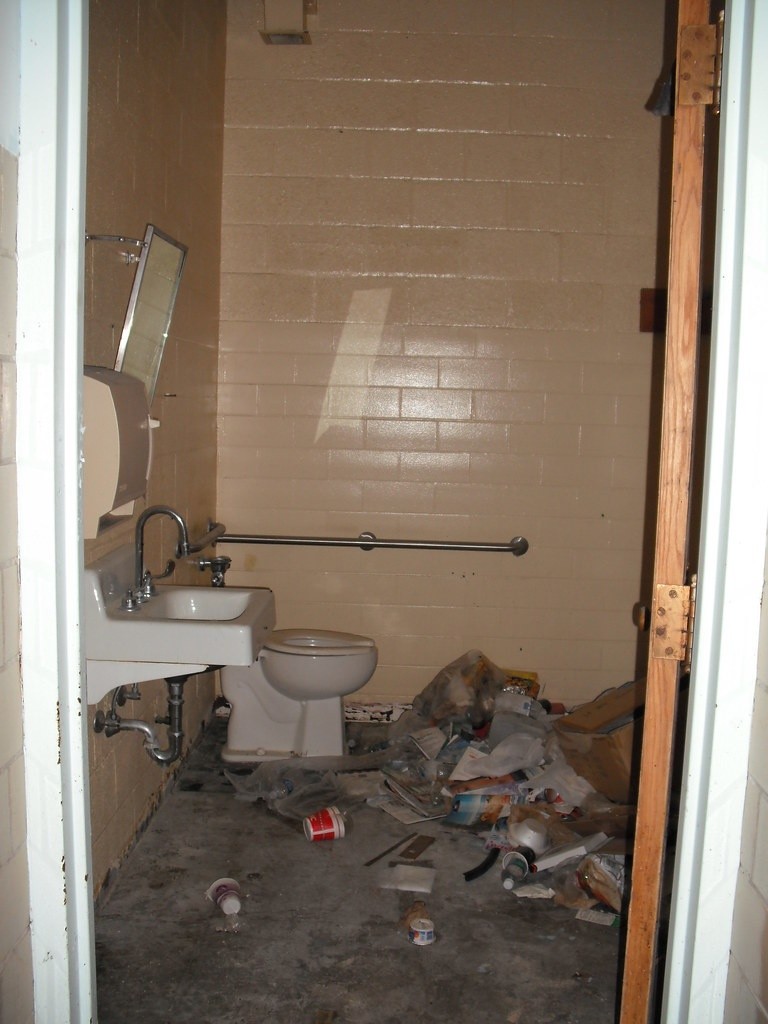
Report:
112,223,189,405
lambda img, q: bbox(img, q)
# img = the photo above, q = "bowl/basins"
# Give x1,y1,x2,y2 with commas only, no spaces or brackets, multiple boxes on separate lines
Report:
510,818,549,849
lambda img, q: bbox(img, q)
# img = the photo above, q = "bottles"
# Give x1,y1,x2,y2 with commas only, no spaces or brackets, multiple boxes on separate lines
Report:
270,774,294,799
385,764,424,791
503,856,525,889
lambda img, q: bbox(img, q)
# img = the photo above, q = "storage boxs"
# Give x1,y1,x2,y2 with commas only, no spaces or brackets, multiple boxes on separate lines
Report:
555,681,634,803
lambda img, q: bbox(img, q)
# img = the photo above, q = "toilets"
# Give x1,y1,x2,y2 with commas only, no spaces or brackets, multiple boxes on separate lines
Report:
218,629,378,764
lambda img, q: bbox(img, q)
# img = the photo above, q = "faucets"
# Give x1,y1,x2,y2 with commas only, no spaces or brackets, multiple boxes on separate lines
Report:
134,504,188,586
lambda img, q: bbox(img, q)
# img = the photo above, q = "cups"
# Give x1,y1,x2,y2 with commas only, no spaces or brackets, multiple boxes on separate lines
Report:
503,845,536,880
209,877,242,915
304,806,345,841
409,918,436,944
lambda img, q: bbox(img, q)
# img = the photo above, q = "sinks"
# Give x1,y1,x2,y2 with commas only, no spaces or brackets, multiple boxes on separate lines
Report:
86,544,277,708
